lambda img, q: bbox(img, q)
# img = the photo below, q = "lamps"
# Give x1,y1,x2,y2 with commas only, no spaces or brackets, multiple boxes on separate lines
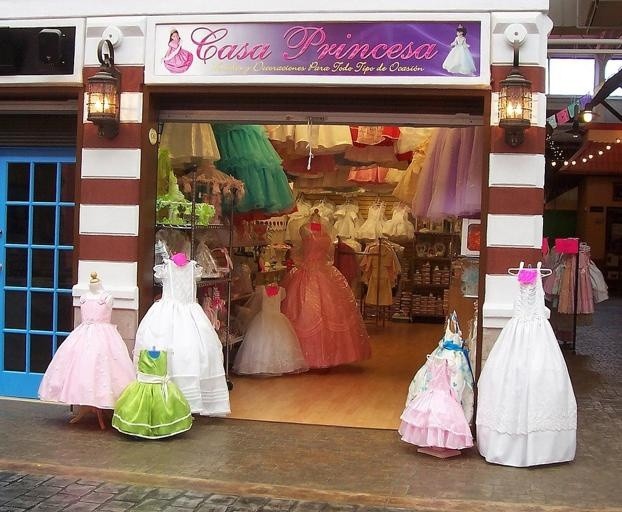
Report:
85,39,121,139
583,103,593,122
497,40,534,147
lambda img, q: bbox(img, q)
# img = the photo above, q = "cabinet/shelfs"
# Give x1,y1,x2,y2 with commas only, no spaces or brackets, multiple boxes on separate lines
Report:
158,164,238,390
394,222,464,322
231,211,289,344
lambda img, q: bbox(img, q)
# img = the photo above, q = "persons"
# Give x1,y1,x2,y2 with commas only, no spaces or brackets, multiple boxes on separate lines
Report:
442,28,477,75
162,29,193,73
132,253,232,419
37,280,137,410
111,350,193,439
278,221,371,371
233,283,313,377
